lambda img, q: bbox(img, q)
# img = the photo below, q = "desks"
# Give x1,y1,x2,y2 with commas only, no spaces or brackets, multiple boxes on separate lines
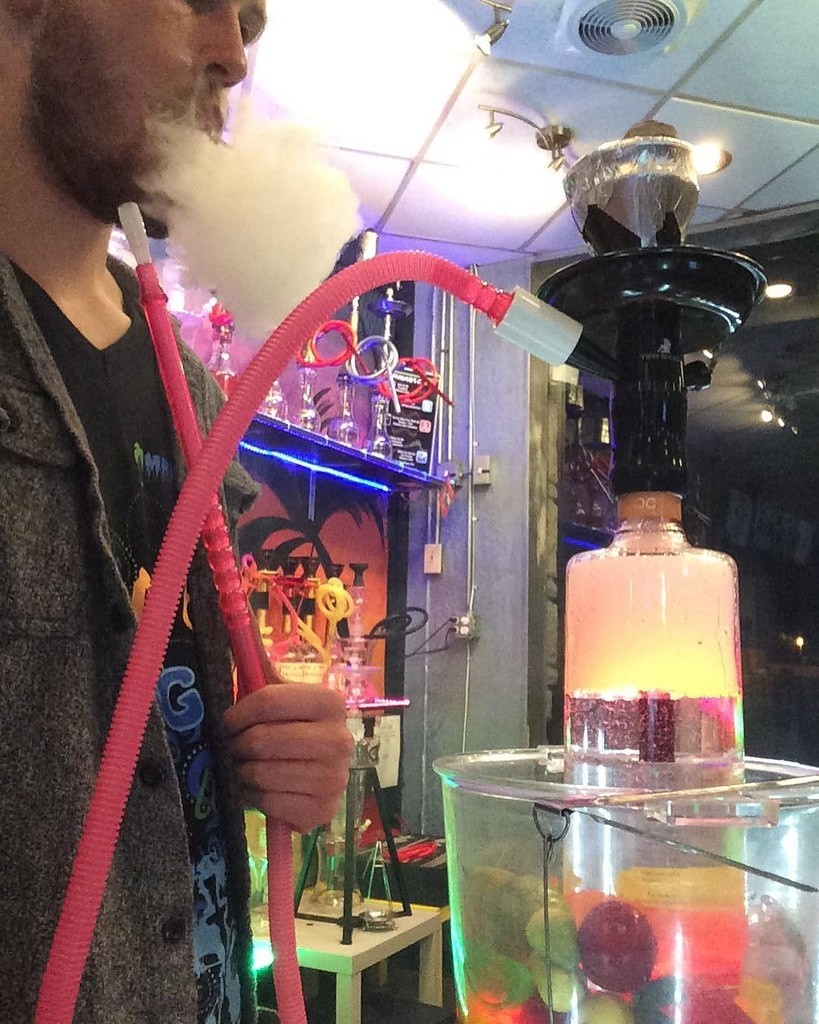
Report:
246,888,444,1024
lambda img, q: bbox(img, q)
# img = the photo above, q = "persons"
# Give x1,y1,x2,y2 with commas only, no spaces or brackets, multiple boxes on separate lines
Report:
0,0,355,1024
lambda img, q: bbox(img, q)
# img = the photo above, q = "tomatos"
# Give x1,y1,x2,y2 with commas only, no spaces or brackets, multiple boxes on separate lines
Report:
575,900,658,994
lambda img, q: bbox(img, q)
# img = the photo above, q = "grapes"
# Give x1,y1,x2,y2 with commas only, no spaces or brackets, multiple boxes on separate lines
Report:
524,908,637,1023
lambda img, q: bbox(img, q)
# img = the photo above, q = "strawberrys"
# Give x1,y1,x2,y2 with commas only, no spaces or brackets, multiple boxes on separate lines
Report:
632,974,754,1023
